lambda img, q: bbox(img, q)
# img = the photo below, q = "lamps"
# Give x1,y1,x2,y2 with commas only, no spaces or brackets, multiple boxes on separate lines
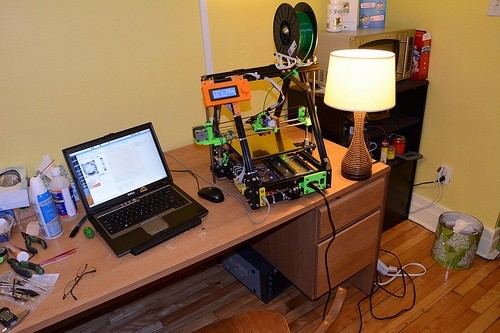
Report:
323,49,397,182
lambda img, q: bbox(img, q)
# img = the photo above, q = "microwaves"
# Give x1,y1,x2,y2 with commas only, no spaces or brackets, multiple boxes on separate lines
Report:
307,24,416,90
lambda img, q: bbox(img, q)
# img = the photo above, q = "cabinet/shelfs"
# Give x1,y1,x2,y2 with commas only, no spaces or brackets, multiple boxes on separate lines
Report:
288,79,429,233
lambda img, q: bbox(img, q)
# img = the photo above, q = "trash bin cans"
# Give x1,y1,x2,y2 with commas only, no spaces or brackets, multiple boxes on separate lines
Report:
431,210,484,270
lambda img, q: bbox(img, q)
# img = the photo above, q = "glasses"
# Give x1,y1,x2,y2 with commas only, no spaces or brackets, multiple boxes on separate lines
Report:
63,263,97,301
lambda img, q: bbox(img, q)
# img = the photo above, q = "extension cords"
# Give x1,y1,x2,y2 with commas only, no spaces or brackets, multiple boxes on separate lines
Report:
377,259,389,275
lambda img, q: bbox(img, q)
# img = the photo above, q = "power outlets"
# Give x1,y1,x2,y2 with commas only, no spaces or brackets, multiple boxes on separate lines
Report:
436,165,453,186
487,0,500,17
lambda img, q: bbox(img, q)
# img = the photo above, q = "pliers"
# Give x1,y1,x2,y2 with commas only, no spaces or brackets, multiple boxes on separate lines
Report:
21,231,47,253
7,257,44,278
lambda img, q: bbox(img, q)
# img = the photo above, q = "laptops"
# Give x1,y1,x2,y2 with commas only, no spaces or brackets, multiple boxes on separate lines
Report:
62,122,209,257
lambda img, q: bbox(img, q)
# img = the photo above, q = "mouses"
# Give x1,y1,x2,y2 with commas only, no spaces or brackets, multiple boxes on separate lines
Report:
198,187,224,203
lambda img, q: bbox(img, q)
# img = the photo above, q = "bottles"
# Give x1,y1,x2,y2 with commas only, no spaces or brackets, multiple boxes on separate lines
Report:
379,138,389,164
69,179,80,202
48,167,79,222
327,0,344,33
29,175,63,239
59,164,69,187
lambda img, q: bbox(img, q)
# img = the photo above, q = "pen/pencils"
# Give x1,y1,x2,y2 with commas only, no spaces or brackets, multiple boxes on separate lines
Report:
69,212,87,237
38,246,82,267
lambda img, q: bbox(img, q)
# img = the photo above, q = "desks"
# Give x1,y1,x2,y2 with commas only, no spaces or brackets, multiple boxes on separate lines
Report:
1,119,392,333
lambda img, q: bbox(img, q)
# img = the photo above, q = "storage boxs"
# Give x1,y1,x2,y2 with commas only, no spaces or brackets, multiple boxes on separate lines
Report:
341,0,432,81
222,247,293,305
